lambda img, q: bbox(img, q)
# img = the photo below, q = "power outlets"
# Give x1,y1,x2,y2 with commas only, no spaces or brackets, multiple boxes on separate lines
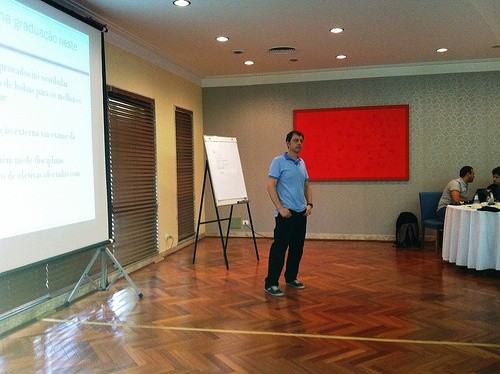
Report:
242,220,249,225
165,234,171,240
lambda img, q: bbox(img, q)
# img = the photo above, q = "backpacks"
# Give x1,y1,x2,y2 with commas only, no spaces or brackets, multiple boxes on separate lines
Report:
396,212,421,248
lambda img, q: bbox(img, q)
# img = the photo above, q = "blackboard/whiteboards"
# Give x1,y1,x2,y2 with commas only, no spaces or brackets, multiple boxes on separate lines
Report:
202,135,250,207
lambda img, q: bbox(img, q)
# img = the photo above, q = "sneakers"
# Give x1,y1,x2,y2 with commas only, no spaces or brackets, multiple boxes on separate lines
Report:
287,280,305,289
264,286,284,296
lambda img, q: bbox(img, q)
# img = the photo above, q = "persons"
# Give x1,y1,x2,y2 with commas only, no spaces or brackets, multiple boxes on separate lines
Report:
264,131,313,298
487,167,500,202
438,166,476,223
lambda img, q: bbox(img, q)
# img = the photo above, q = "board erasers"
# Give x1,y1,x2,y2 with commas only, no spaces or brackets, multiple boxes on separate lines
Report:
237,201,247,203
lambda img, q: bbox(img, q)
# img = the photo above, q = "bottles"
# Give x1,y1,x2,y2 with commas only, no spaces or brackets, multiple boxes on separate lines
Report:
474,193,479,204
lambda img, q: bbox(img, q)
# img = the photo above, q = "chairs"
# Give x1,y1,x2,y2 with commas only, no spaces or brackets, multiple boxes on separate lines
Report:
419,191,444,257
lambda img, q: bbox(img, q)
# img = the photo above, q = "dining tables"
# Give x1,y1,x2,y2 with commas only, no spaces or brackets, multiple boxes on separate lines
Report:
441,202,500,275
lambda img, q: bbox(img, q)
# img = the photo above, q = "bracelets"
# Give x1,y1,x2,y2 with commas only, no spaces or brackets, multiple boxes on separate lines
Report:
307,203,313,209
